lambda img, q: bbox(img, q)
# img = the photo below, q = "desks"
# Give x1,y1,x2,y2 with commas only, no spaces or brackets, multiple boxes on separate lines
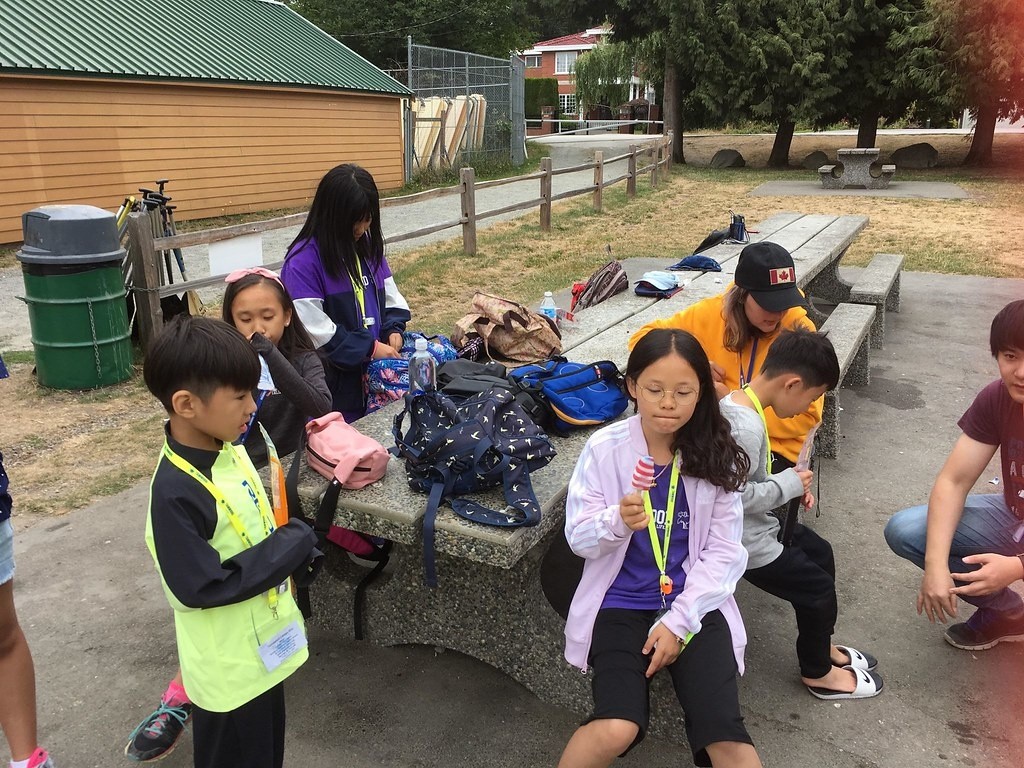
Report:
256,211,870,717
836,147,882,190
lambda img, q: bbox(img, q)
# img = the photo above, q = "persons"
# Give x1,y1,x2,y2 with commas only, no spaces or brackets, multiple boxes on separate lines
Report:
124,267,332,762
629,242,824,513
280,165,411,427
884,297,1024,650
557,329,763,768
709,325,884,700
143,316,318,768
0,353,53,768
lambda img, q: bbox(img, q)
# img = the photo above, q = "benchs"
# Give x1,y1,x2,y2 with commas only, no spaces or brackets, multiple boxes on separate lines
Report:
814,254,906,461
882,164,896,189
818,165,837,189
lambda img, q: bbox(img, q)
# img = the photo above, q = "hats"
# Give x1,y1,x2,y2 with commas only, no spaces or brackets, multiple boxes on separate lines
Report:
734,241,809,311
635,270,677,291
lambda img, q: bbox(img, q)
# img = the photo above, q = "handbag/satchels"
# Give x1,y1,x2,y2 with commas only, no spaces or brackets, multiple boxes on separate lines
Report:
361,332,459,413
301,411,390,492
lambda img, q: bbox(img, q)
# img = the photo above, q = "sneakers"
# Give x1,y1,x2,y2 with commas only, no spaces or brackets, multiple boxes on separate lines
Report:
944,606,1024,649
124,693,193,764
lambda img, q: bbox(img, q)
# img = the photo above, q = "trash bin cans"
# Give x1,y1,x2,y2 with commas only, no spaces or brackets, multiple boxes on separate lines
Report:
15,205,134,390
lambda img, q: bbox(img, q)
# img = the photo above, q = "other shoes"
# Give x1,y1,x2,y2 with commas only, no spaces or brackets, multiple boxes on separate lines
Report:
7,747,53,768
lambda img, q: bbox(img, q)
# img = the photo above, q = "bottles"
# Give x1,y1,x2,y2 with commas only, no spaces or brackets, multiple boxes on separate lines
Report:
408,339,436,396
490,361,495,365
730,215,744,243
540,292,556,324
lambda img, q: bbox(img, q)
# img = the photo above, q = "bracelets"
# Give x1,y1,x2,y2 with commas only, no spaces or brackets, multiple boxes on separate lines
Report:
675,635,686,644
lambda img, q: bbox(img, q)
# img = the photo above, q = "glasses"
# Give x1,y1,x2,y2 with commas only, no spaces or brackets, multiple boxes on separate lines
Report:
634,380,700,406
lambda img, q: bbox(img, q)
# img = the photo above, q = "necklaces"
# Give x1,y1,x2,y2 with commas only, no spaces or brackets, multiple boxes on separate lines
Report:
651,451,677,487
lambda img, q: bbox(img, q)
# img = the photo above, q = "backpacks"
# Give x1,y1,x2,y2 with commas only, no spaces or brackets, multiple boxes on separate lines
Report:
392,350,632,529
571,260,629,316
449,291,565,367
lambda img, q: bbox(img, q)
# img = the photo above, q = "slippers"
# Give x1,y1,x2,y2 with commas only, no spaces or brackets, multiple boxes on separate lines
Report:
808,666,884,701
831,645,878,671
